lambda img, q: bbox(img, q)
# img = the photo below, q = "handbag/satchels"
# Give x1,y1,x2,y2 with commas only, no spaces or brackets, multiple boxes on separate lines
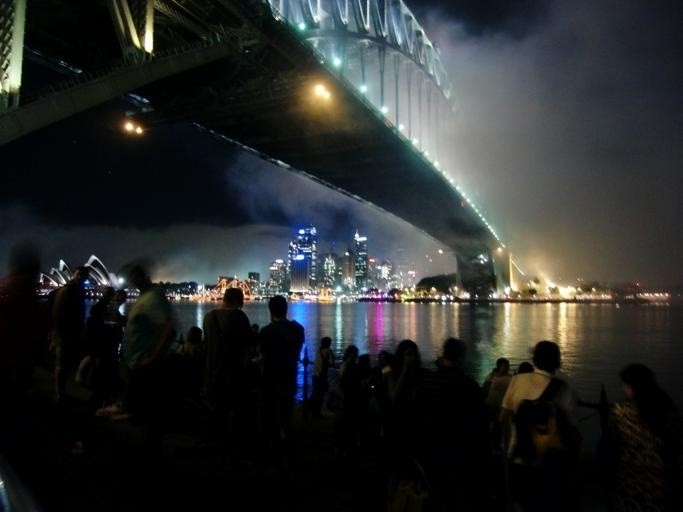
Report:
320,349,329,375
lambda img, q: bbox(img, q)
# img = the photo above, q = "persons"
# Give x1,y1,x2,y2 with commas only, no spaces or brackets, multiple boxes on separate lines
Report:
499,337,570,472
50,266,89,402
120,266,172,440
309,335,337,412
601,364,681,508
484,358,510,419
176,326,202,359
203,286,252,368
258,294,303,411
